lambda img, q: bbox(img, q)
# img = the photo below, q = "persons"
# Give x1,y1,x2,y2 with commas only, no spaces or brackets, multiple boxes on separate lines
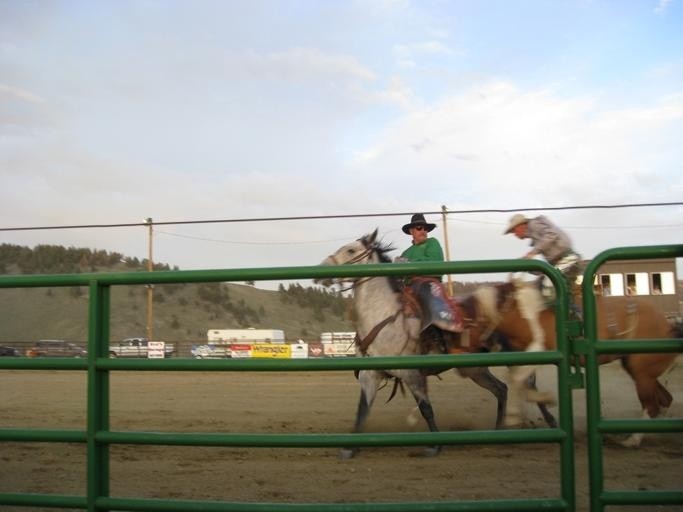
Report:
503,214,584,327
394,214,464,354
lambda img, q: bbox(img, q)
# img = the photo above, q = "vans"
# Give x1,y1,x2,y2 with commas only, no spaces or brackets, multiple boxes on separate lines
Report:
31,339,88,359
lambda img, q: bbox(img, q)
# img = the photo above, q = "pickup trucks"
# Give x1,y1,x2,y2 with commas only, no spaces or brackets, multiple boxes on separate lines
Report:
108,337,176,360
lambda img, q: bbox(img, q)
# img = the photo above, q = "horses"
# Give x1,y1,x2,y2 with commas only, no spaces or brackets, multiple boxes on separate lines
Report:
453,280,683,450
309,226,559,459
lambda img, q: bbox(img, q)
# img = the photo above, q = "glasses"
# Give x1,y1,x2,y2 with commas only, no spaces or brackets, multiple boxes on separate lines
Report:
416,226,428,231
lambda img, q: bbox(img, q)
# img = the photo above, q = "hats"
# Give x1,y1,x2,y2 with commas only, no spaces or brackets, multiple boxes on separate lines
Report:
402,214,436,235
504,214,530,234
